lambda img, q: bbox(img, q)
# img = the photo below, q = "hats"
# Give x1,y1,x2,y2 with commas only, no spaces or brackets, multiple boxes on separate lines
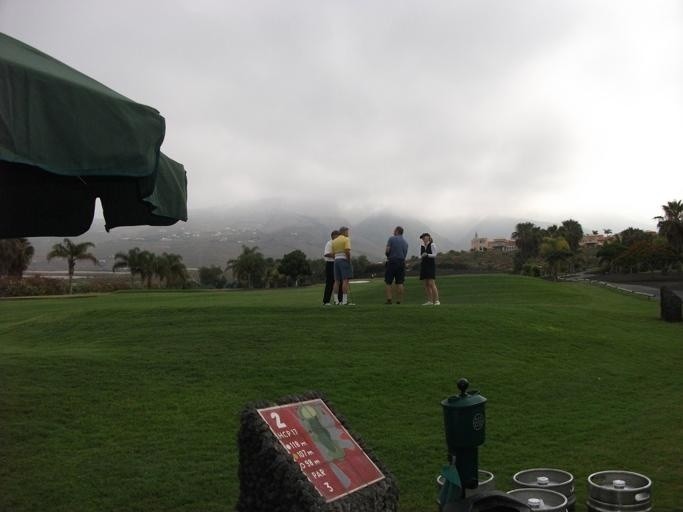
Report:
419,232,432,238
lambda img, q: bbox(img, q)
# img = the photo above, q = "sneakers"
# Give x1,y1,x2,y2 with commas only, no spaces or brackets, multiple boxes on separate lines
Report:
322,301,356,307
396,300,400,304
385,298,393,304
421,300,441,306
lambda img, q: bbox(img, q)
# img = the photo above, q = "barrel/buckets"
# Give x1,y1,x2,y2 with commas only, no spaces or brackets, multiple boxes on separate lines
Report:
506,487,569,512
436,469,493,508
512,468,576,512
585,470,653,512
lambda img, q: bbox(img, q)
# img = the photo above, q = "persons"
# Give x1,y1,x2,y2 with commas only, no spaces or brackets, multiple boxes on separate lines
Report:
385,226,408,305
322,226,355,306
418,233,440,306
